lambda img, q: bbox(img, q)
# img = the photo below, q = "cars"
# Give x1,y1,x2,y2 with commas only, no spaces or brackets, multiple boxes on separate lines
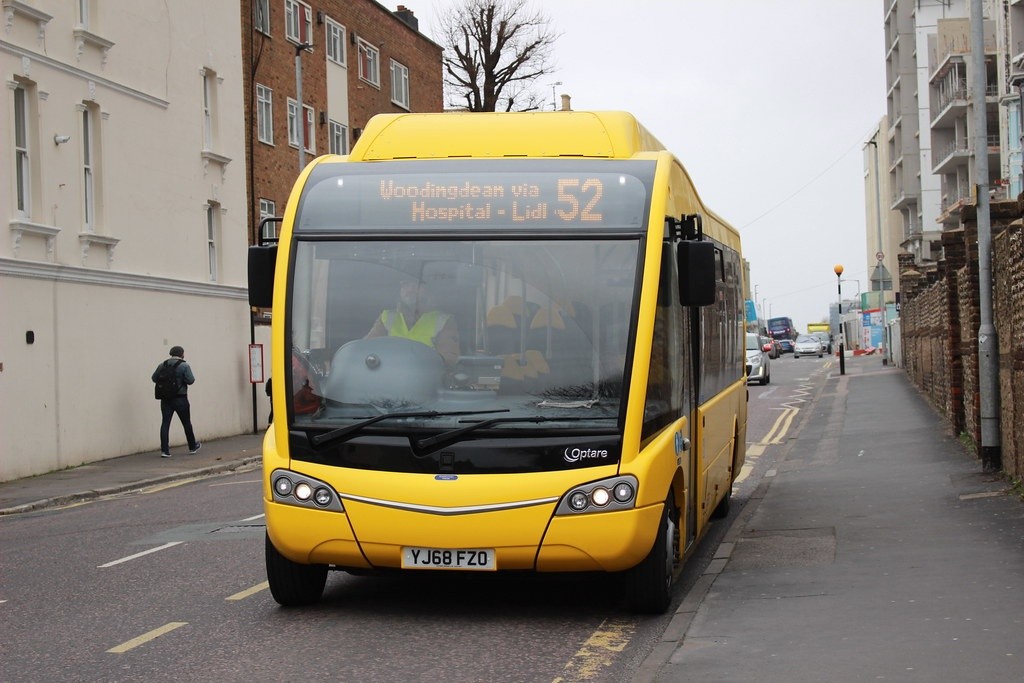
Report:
746,333,771,386
794,335,824,359
761,336,795,359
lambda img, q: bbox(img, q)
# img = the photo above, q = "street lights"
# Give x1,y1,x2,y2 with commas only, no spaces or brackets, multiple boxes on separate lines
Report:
834,265,845,375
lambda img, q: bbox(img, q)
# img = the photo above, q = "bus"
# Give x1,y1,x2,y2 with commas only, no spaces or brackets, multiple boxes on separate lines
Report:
767,317,797,340
260,111,749,616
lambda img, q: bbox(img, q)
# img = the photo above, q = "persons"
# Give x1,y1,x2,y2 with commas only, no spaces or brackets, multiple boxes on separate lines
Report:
265,377,274,432
362,266,461,370
151,346,202,458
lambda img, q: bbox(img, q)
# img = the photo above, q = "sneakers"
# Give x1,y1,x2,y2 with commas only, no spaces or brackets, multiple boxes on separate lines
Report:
189,442,201,454
161,451,172,457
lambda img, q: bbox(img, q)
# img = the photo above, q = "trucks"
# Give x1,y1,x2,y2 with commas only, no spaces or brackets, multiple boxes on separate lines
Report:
807,322,832,354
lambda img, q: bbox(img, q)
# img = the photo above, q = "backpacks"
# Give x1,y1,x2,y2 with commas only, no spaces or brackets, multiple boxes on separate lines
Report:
154,360,186,400
